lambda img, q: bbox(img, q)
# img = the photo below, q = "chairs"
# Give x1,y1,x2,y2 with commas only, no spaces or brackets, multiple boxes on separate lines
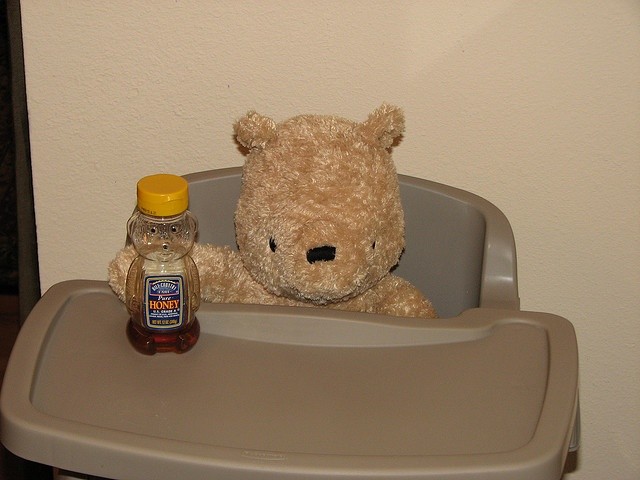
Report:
122,161,519,316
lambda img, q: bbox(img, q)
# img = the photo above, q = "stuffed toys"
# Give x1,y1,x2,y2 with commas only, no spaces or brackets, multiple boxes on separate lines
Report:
107,102,438,318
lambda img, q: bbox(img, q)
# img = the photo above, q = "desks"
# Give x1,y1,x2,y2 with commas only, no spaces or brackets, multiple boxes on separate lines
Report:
2,277,578,474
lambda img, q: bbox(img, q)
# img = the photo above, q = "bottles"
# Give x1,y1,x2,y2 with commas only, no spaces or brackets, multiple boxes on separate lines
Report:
125,174,201,356
